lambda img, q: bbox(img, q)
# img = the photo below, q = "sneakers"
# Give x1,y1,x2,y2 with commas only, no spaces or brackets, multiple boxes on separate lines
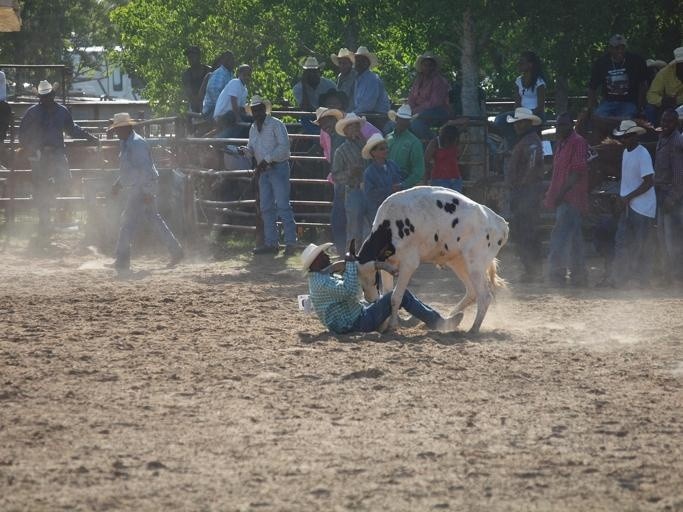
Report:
252,240,296,256
510,267,681,290
434,311,465,331
103,248,183,268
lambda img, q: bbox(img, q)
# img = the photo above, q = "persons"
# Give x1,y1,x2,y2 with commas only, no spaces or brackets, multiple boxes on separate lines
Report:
300,241,464,333
103,112,183,270
19,81,98,229
489,35,683,287
183,46,464,256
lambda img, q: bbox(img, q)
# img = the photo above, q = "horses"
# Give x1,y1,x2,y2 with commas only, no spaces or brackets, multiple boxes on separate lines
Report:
574,107,663,179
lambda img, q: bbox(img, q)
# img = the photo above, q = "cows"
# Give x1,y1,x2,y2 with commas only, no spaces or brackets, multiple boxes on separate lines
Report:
0,145,98,168
350,185,512,338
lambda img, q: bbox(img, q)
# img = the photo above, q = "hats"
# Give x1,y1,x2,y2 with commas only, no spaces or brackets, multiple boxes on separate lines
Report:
413,50,443,72
244,93,272,116
504,105,542,128
611,119,646,139
300,240,333,275
308,87,419,159
607,34,682,67
106,112,138,133
298,44,378,75
37,80,53,95
